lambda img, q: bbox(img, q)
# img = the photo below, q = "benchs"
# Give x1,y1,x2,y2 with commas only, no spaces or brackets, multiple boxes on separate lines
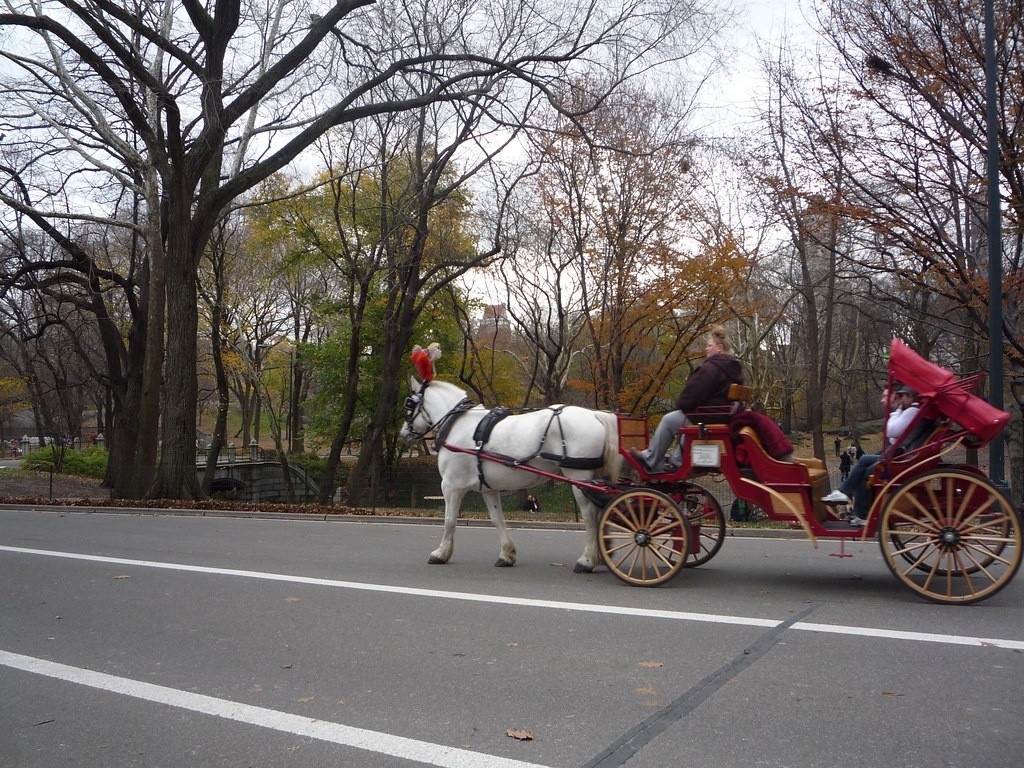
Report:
740,425,827,487
866,424,948,489
678,384,753,438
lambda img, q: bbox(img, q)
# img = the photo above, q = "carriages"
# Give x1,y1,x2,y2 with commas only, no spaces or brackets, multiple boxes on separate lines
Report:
396,337,1023,607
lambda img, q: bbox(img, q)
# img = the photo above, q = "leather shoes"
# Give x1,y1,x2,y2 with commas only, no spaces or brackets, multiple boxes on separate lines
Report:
662,457,678,473
630,447,654,474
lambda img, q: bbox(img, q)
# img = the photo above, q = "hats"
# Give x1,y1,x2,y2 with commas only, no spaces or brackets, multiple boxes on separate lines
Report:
896,385,916,396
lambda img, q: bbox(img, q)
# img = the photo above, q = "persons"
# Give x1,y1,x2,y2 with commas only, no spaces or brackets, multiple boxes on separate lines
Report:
730,497,752,521
523,494,540,512
632,325,746,473
819,383,929,526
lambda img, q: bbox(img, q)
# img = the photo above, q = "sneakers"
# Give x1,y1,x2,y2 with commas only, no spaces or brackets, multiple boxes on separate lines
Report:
849,517,868,527
819,488,849,505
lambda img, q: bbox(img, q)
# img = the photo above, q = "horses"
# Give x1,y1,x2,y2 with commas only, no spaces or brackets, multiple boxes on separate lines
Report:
401,374,620,576
